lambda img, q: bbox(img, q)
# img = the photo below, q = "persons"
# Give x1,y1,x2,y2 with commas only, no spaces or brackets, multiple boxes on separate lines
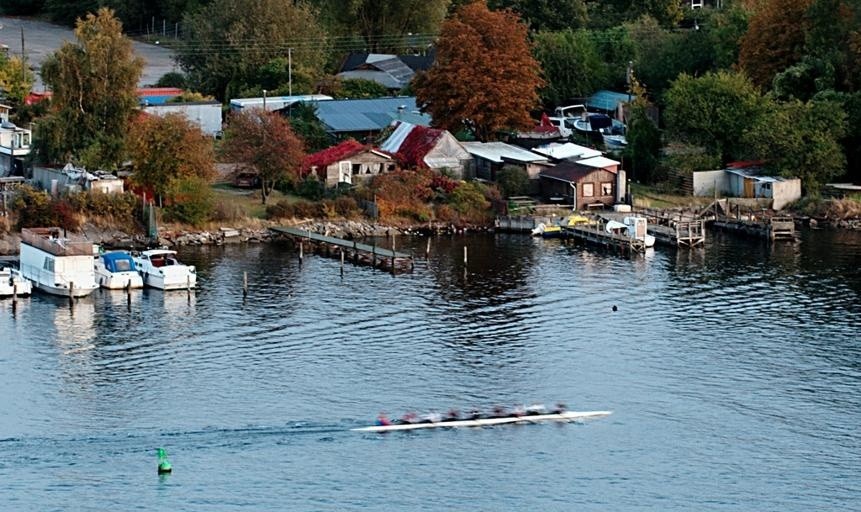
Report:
376,402,570,425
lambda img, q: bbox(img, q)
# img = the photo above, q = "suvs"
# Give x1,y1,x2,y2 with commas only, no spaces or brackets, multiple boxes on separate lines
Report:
539,117,574,142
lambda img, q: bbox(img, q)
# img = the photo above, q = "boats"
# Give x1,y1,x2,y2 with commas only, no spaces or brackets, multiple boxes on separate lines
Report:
540,215,599,237
606,217,656,247
0,289,196,332
350,410,612,431
9,226,100,297
0,271,33,297
93,252,143,290
131,249,197,291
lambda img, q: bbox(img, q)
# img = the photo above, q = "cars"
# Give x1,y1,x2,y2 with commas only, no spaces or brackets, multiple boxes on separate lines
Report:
554,102,588,118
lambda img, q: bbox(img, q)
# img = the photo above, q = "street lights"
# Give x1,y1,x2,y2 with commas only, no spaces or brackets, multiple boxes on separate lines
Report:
288,46,299,106
